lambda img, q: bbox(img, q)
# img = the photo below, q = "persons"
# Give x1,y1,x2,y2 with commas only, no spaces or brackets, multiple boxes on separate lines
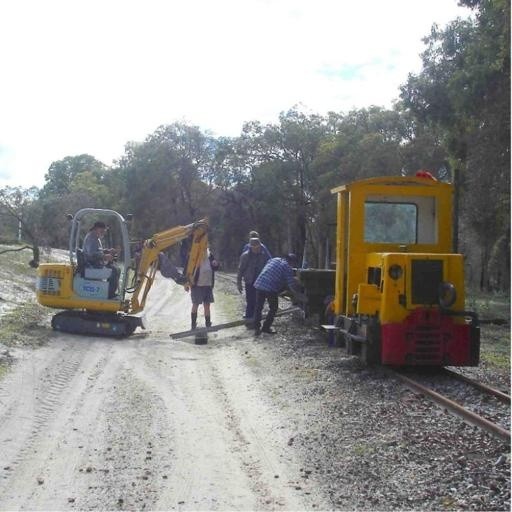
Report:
237,237,270,329
242,231,272,260
83,220,122,300
252,253,299,335
185,241,219,330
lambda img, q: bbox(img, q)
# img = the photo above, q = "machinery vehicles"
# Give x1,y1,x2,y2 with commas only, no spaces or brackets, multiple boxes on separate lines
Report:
32,207,213,341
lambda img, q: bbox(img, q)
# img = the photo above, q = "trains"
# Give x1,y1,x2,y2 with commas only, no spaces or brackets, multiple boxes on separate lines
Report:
289,165,484,376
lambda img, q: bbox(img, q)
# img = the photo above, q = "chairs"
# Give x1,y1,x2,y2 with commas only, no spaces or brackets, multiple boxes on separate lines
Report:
76,248,112,280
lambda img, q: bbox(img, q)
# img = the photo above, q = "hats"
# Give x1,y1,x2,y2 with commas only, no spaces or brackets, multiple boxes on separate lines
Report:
246,239,264,249
95,221,110,231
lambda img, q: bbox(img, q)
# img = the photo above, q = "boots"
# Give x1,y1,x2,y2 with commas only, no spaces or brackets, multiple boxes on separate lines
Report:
204,314,212,327
242,315,277,336
190,311,198,329
